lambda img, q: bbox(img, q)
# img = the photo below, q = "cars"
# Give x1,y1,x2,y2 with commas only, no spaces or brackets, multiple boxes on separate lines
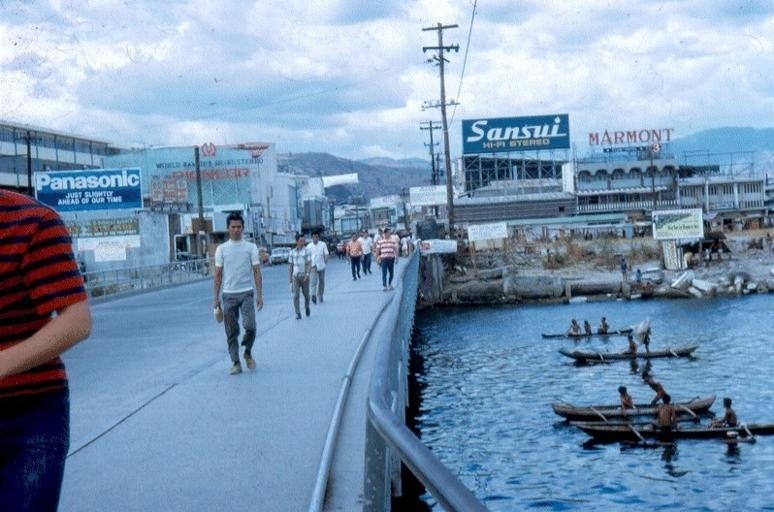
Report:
336,239,352,254
270,246,292,264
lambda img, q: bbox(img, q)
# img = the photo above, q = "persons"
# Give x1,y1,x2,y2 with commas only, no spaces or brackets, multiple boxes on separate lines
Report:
0,188,92,512
346,228,408,290
636,269,643,285
702,247,712,266
618,328,677,430
598,317,609,334
707,398,737,429
584,320,591,336
305,231,329,303
288,233,312,319
213,212,263,374
620,258,632,281
564,319,581,336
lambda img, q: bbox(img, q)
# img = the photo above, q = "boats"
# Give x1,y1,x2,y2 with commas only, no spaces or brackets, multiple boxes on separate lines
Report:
571,419,773,442
558,345,699,362
548,395,717,421
540,325,636,339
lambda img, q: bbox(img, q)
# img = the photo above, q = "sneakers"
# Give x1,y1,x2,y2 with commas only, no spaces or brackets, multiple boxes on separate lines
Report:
318,295,321,302
311,295,314,301
294,312,299,318
383,284,395,292
230,362,239,376
305,307,308,315
350,268,372,281
245,358,253,368
